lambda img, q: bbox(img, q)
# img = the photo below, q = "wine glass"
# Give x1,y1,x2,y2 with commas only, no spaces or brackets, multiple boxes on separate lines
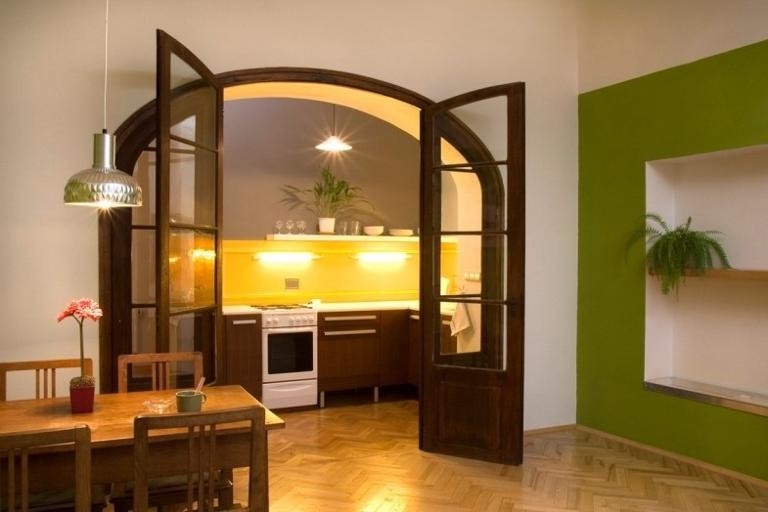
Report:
275,219,306,235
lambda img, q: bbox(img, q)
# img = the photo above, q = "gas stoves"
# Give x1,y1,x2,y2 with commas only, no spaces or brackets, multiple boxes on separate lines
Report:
251,303,317,312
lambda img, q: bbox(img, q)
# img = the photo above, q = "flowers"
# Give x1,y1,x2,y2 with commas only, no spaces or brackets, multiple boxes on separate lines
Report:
56,296,103,380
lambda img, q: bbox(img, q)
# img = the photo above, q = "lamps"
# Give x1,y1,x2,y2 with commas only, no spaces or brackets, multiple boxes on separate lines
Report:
63,2,144,208
315,105,353,152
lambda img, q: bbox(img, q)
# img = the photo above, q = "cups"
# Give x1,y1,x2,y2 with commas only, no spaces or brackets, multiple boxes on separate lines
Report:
338,220,359,235
175,390,207,413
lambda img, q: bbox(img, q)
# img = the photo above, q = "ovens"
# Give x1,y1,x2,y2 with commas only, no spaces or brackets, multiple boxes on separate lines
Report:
261,312,319,410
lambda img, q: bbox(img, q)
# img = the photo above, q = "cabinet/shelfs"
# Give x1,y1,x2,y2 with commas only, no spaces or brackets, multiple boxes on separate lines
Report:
643,143,768,418
223,310,457,412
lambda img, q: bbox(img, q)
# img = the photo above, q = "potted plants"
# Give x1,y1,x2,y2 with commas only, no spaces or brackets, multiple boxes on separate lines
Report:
272,164,376,232
620,213,736,303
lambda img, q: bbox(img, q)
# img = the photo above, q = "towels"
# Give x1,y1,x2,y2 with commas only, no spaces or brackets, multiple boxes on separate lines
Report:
450,291,471,337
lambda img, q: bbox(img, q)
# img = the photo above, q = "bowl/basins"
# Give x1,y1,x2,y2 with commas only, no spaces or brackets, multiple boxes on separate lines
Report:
389,229,414,236
364,226,384,235
